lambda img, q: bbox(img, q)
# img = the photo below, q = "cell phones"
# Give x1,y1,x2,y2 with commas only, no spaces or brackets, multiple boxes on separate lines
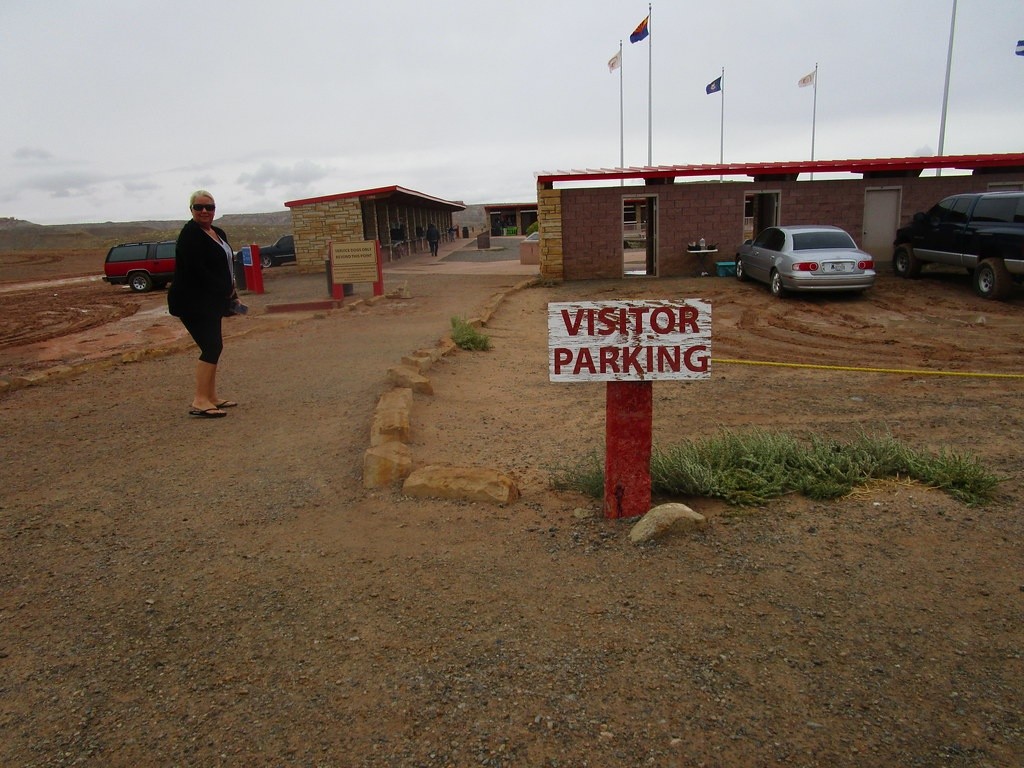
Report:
230,300,248,316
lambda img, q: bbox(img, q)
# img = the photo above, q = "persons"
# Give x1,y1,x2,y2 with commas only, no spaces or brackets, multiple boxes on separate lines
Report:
426,224,440,257
175,190,243,417
448,227,455,242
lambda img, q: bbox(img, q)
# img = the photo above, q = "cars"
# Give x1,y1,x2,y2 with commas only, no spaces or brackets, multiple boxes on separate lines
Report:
523,230,539,241
736,224,876,298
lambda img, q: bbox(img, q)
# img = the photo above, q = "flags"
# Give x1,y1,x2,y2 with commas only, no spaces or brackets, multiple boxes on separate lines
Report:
608,50,622,73
706,75,724,94
630,14,651,43
798,69,817,88
1015,40,1024,56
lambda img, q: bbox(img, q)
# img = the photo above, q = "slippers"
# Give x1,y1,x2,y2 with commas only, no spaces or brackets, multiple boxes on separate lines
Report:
217,401,237,407
189,407,227,417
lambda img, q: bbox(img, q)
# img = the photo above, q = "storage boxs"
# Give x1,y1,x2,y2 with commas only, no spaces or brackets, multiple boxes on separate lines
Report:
714,261,737,277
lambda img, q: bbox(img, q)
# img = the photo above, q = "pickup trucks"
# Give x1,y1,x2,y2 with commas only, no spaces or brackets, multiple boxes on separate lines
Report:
893,191,1024,301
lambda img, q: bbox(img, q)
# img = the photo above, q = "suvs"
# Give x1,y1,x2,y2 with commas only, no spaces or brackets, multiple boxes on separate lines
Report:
257,234,296,268
102,240,179,293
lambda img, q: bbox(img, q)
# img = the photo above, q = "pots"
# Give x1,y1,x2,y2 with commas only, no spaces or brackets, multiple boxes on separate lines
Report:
707,243,719,250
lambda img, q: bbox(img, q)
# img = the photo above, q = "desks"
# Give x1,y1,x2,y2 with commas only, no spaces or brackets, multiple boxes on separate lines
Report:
686,248,719,277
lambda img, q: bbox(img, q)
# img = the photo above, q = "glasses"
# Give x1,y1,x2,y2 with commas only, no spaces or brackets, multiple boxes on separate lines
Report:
192,204,215,211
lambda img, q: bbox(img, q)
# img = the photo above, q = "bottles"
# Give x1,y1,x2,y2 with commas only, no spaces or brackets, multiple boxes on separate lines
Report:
700,238,705,250
